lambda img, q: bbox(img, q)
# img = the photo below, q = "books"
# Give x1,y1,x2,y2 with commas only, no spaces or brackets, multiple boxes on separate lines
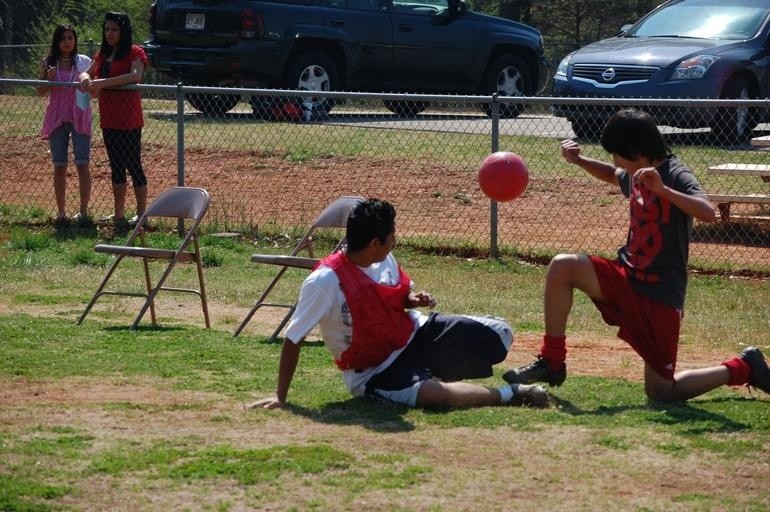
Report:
74,88,91,111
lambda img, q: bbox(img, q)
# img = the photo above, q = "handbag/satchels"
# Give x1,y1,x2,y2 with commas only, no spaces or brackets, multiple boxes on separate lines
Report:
250,95,307,122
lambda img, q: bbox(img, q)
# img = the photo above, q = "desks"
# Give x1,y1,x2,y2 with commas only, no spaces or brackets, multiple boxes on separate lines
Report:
752,135,770,147
707,162,770,183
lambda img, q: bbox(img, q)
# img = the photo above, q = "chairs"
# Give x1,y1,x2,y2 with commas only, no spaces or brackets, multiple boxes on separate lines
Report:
231,195,373,345
73,187,214,331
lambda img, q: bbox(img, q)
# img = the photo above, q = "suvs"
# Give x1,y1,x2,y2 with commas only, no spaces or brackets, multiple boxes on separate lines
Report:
140,0,550,121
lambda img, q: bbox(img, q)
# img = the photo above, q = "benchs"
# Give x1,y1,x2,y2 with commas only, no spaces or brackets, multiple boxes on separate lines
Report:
688,190,769,237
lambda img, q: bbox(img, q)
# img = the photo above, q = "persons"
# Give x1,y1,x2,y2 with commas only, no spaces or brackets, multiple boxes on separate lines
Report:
78,9,160,233
38,22,95,227
246,197,552,413
501,107,769,405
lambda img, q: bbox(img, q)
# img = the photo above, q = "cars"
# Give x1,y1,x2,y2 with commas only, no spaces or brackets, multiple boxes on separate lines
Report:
549,0,770,146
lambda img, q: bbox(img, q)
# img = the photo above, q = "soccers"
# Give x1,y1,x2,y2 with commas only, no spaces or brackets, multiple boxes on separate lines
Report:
478,151,529,201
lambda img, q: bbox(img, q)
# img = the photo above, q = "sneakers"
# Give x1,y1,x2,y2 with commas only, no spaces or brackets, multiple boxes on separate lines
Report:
509,383,550,405
740,347,770,396
128,214,151,225
502,357,566,388
70,212,88,219
100,213,125,221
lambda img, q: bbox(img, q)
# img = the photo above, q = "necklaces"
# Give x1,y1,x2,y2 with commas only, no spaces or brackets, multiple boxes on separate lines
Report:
55,55,76,89
